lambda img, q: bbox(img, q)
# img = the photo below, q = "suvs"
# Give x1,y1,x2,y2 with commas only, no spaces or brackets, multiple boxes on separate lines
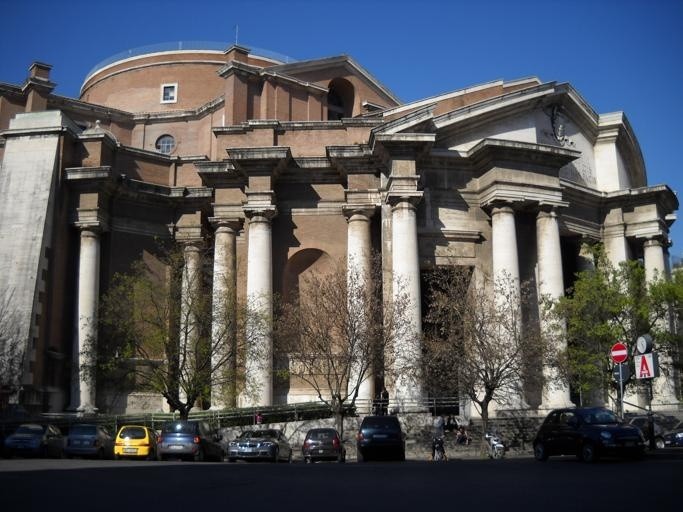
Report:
4,423,64,456
158,420,226,461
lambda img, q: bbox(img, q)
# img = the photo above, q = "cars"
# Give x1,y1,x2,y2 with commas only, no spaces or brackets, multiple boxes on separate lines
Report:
64,423,115,459
113,423,161,460
228,429,292,460
530,406,646,460
627,413,682,448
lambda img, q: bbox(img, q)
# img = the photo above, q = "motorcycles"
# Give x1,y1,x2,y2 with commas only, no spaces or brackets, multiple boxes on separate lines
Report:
432,435,447,463
483,431,504,458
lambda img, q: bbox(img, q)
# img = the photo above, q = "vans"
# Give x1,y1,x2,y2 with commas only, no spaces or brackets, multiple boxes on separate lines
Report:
354,415,407,462
303,427,346,465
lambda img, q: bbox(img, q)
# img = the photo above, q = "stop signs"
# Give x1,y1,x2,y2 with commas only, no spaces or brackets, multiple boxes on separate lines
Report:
609,343,627,362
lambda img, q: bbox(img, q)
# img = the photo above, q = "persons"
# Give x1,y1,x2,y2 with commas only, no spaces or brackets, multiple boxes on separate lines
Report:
435,414,445,439
445,413,459,433
256,413,264,424
380,388,389,416
371,393,380,416
455,427,470,445
431,438,447,463
